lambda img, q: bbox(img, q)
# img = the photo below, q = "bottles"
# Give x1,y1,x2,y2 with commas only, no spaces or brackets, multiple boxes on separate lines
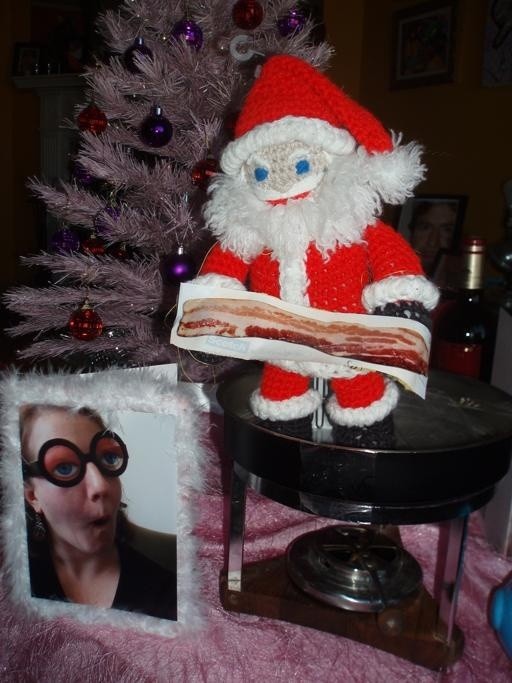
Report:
436,236,500,379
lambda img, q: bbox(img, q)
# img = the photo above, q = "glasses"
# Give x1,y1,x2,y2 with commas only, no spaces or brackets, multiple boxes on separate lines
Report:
23,430,127,487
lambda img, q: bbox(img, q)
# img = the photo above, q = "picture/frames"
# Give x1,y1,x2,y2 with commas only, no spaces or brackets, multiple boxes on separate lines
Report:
393,192,467,270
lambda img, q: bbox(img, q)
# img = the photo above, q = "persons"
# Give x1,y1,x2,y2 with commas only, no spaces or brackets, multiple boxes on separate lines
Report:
173,54,441,431
19,400,178,623
409,201,459,262
